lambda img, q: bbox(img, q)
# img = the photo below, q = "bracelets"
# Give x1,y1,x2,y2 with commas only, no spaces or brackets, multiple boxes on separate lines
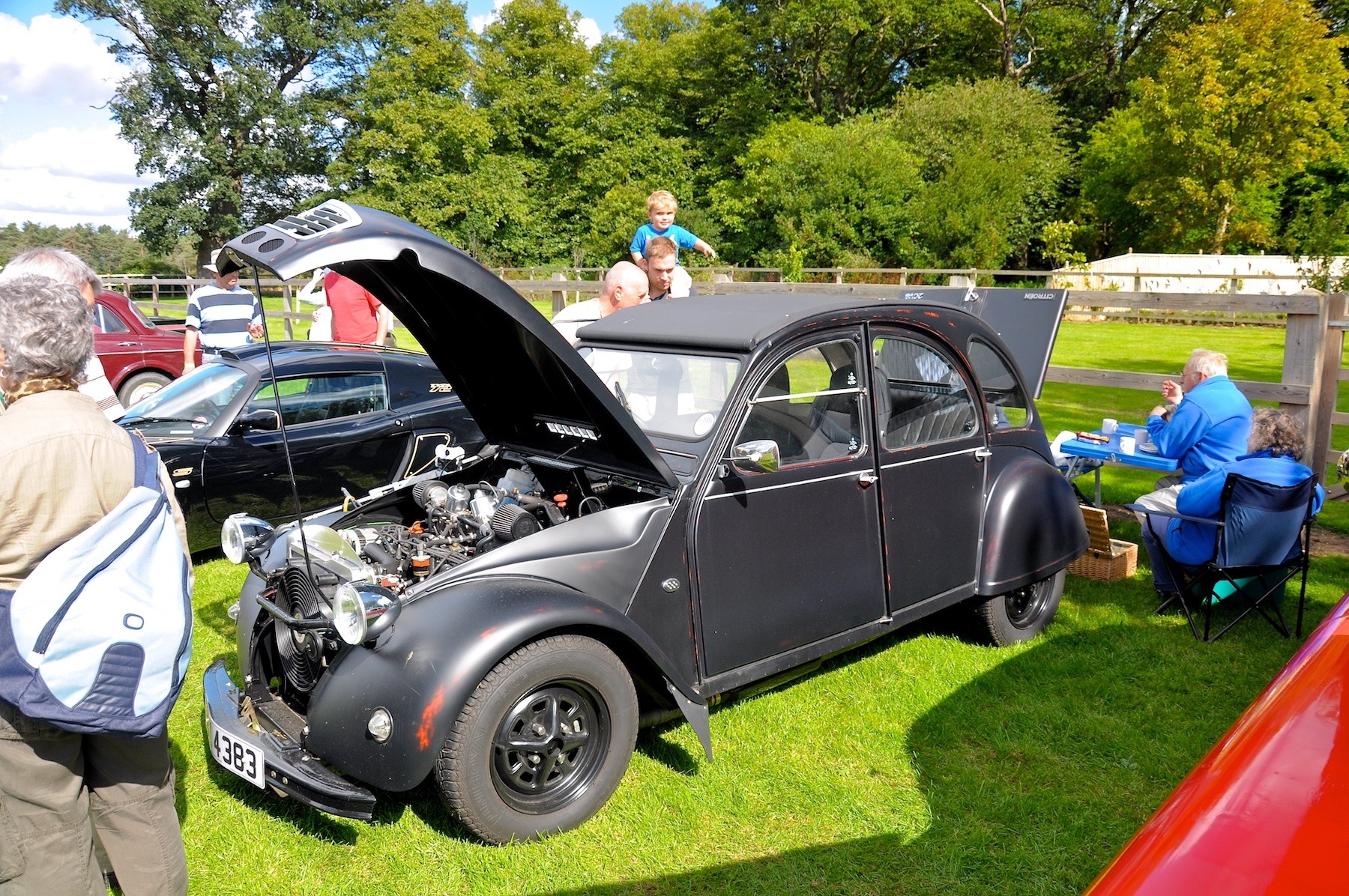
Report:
184,363,195,368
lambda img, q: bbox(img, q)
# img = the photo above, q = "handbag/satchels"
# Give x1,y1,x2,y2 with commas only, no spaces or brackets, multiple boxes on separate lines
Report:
3,434,194,739
1051,430,1101,467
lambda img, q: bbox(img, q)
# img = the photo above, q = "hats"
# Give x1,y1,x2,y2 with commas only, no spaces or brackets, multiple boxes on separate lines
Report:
202,249,222,272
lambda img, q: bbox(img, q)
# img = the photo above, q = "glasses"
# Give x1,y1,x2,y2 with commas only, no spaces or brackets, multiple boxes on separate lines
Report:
1180,372,1207,380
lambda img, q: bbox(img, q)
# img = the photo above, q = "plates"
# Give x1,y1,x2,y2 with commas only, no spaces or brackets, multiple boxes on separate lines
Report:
1139,443,1158,454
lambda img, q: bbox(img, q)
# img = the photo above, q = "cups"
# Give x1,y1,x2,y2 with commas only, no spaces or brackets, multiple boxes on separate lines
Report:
1119,437,1136,455
1134,429,1152,445
1102,418,1119,434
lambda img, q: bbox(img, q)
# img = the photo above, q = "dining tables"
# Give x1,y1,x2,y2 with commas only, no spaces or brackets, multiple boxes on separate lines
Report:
1060,423,1183,510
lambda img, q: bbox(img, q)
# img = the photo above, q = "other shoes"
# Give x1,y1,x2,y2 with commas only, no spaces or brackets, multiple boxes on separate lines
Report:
1152,588,1184,614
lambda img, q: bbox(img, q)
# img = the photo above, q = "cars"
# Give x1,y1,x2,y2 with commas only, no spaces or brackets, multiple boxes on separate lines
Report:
200,198,1093,847
91,288,203,409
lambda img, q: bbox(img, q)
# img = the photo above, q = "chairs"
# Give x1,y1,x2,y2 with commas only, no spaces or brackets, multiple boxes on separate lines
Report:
734,364,999,472
1123,472,1320,645
1055,458,1104,509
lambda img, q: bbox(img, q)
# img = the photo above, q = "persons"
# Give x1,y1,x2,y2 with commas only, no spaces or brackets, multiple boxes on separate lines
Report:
182,249,267,406
2,245,125,424
297,267,332,342
1133,348,1256,526
376,303,397,349
629,190,717,422
1,270,193,896
324,266,389,347
1141,405,1327,601
547,261,649,404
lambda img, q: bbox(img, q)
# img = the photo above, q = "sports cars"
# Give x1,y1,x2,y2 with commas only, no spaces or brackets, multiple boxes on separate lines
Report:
113,339,489,515
